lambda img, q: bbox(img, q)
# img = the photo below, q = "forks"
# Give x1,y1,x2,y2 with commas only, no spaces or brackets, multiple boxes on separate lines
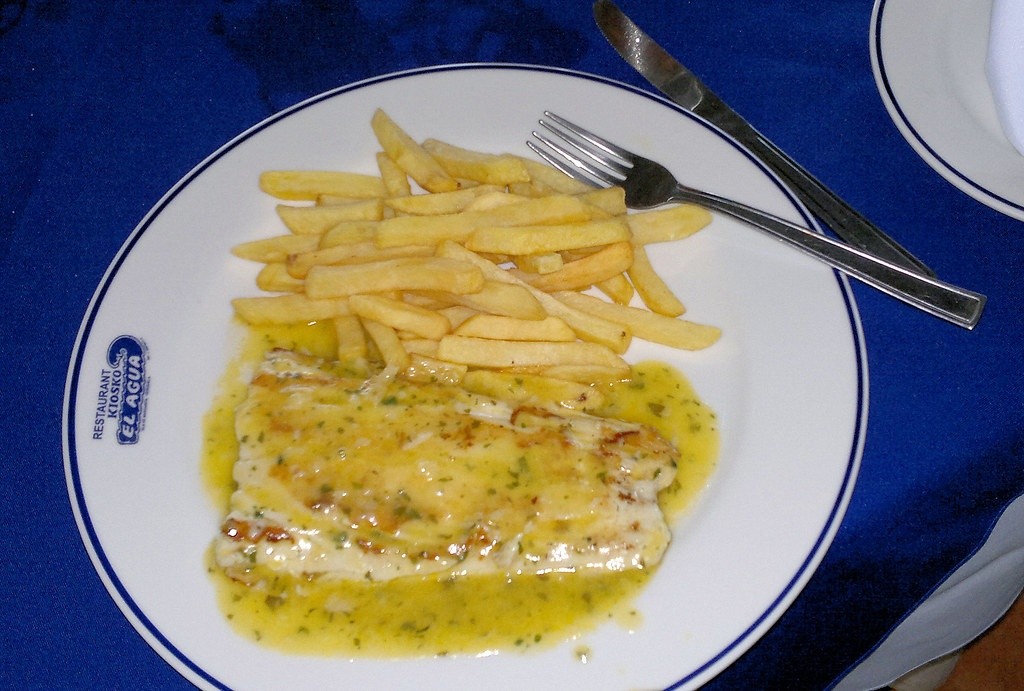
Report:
524,109,988,331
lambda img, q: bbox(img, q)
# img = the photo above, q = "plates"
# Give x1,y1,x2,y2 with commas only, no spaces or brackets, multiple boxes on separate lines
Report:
60,60,870,691
868,1,1024,222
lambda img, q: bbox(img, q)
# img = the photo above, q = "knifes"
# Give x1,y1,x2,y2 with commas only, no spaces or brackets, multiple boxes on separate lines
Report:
592,1,937,281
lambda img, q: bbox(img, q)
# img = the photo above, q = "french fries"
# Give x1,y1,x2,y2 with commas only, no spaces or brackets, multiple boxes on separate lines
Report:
232,105,722,410
